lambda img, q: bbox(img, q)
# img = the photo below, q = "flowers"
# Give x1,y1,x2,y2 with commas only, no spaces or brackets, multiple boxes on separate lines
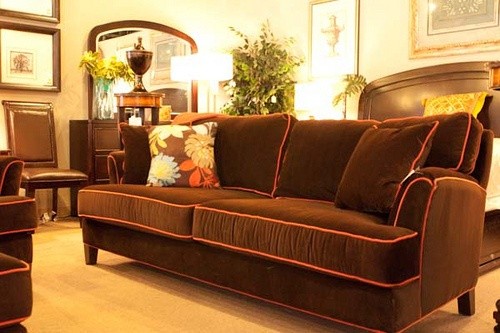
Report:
78,49,136,116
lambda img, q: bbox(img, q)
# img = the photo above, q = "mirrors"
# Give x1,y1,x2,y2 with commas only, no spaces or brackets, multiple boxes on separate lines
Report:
87,19,198,119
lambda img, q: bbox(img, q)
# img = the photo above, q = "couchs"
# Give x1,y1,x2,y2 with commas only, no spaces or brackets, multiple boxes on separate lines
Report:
0,155,38,333
76,114,494,333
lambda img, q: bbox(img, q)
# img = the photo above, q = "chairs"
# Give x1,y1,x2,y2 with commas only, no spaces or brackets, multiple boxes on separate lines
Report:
2,100,89,229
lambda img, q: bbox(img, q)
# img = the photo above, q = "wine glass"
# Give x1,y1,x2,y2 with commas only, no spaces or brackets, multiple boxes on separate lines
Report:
126,50,153,93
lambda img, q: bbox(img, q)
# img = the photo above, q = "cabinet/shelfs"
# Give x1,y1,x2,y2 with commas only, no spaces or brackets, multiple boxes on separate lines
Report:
69,120,171,217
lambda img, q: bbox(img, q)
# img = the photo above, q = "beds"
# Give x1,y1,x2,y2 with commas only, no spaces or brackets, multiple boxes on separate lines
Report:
357,60,500,277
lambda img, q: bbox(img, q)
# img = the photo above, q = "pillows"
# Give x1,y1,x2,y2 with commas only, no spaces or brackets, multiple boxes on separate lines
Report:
422,92,492,119
335,119,440,214
146,121,221,189
119,121,152,184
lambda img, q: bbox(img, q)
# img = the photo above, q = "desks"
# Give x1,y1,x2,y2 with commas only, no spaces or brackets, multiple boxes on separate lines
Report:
114,92,163,150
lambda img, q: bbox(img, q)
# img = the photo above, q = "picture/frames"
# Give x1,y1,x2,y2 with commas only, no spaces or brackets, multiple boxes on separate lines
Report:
0,21,61,92
0,0,61,23
308,0,359,82
409,0,500,60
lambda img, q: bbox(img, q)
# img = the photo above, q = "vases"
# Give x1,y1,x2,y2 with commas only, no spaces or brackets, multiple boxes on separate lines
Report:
91,78,115,119
